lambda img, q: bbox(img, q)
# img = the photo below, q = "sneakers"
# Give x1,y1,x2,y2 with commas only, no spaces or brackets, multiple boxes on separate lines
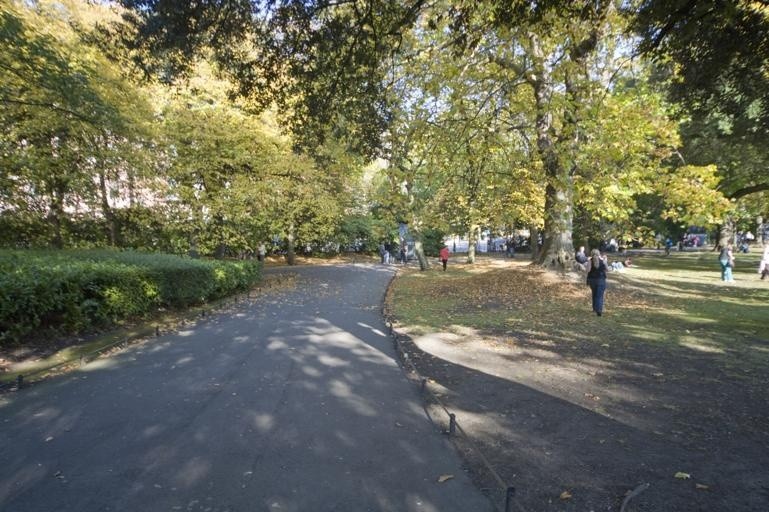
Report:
592,308,602,318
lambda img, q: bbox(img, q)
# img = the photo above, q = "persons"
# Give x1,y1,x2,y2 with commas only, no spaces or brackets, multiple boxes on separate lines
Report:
691,234,699,248
664,234,672,256
611,256,631,269
739,240,749,253
399,235,409,262
379,240,385,262
759,245,769,280
575,245,590,264
509,240,517,258
721,244,737,282
584,248,608,318
439,244,448,272
682,232,688,246
383,241,391,262
